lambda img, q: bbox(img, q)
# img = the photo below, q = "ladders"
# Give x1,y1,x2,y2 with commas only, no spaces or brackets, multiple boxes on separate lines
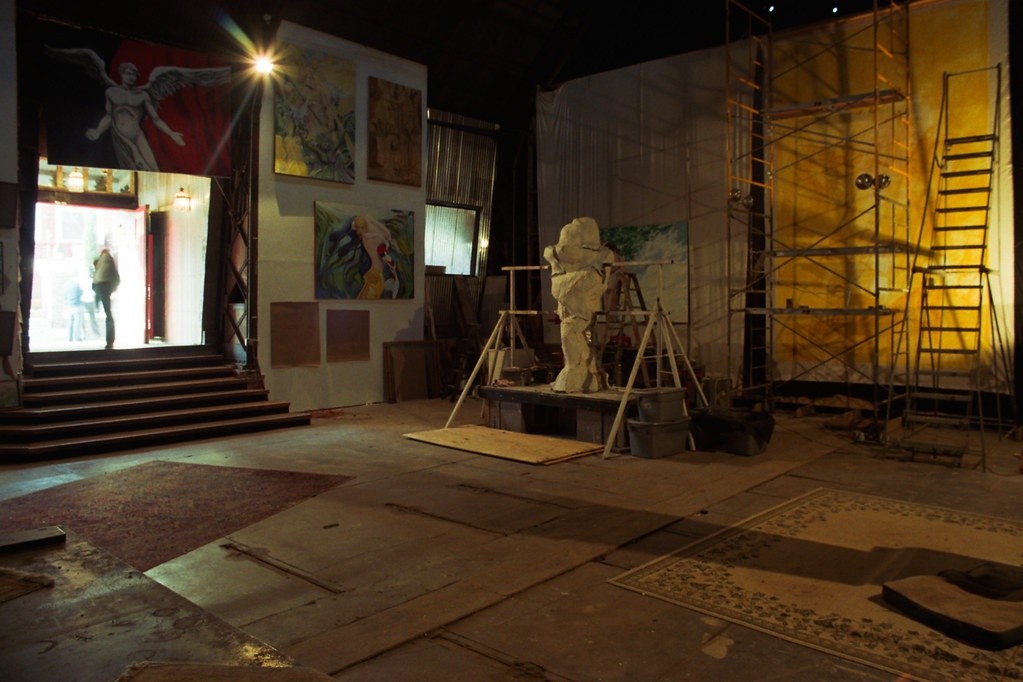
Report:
881,62,1023,474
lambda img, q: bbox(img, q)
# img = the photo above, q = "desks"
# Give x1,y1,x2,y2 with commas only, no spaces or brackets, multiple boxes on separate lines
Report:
478,380,645,449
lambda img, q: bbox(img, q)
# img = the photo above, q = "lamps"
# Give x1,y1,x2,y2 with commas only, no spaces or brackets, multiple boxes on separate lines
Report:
727,187,754,211
856,173,891,193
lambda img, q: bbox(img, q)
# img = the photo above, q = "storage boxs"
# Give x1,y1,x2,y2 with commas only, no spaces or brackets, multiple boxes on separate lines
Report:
627,388,776,460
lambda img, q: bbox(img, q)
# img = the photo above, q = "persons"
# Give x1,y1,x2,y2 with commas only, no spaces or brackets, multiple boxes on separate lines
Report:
67,275,102,341
92,241,120,349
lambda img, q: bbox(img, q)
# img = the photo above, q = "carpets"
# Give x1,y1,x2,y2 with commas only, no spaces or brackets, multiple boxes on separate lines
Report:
0,458,359,578
